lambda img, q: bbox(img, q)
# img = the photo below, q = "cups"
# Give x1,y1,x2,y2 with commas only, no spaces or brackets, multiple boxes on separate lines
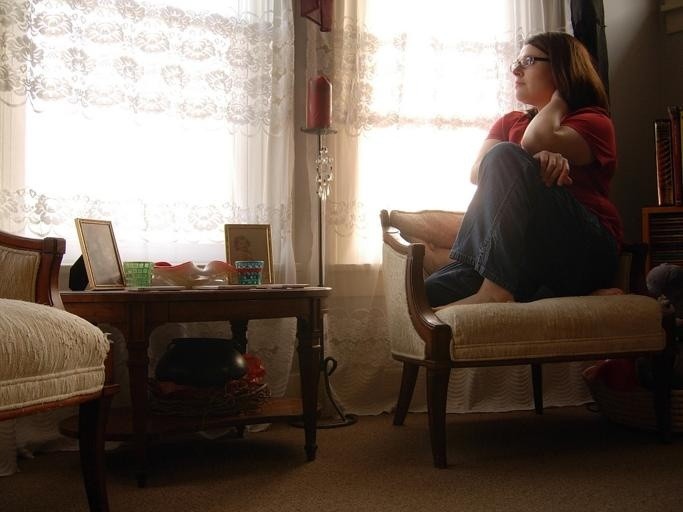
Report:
121,262,154,290
234,261,264,285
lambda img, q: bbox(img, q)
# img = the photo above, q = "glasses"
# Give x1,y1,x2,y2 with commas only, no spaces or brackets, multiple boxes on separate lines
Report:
510,55,549,71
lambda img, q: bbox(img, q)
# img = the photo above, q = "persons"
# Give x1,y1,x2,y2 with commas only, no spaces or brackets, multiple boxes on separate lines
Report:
421,30,628,312
232,235,255,262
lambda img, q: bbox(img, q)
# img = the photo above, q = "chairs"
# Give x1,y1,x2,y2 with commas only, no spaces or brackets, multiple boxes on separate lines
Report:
0,231,121,512
380,210,676,469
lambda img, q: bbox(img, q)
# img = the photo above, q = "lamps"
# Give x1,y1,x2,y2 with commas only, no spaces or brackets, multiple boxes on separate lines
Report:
289,75,359,429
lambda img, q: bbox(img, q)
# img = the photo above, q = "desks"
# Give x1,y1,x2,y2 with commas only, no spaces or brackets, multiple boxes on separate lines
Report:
60,286,333,488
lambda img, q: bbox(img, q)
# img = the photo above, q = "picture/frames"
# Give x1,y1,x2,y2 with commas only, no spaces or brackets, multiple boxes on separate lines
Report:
68,218,126,291
224,223,275,285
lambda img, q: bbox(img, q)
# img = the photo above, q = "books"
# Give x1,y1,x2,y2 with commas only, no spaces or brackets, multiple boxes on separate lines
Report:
648,104,683,273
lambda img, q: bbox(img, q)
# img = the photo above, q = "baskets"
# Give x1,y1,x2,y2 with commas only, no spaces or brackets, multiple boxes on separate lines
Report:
583,364,683,432
